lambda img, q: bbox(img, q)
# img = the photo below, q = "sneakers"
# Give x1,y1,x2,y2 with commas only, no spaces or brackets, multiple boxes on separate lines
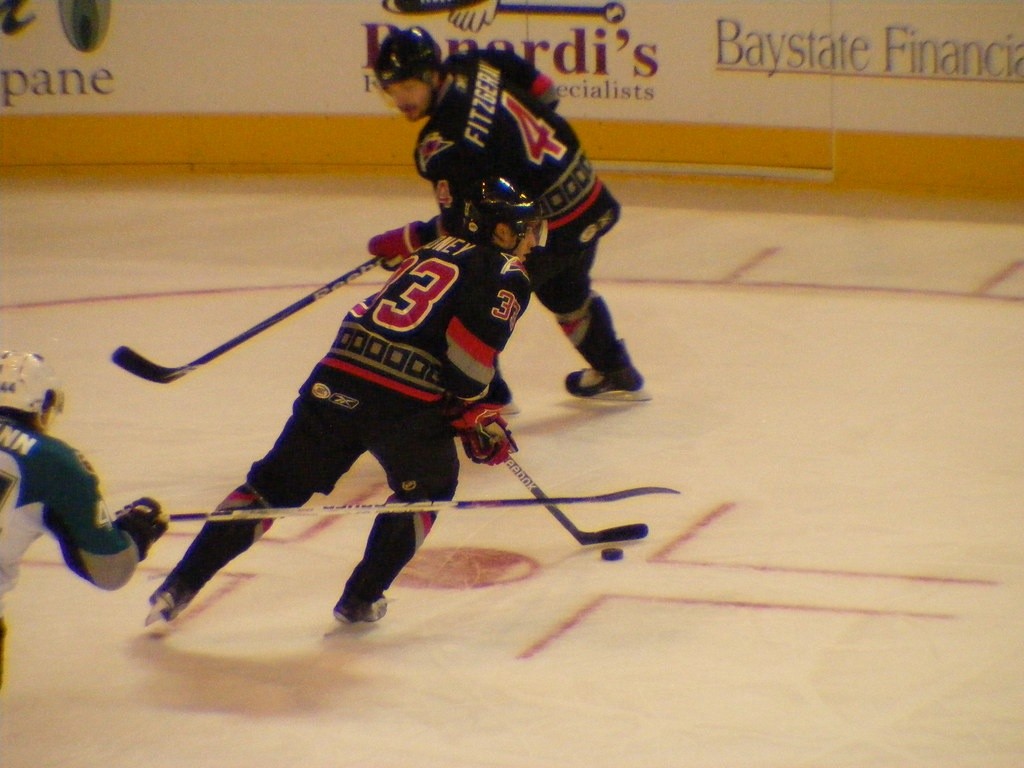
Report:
145,582,185,626
331,596,388,635
565,339,650,400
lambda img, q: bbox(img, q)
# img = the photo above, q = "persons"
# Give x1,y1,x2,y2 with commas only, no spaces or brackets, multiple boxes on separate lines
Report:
369,26,645,408
0,349,171,709
149,175,539,626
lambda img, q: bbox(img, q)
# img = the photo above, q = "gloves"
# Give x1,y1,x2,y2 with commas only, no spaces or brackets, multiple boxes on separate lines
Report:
368,218,425,273
448,402,513,466
113,496,171,561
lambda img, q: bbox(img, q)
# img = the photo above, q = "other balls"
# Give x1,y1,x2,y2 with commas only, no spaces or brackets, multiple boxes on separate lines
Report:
601,548,624,561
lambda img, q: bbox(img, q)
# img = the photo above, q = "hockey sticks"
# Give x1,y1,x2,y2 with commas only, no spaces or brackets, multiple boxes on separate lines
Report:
112,485,685,525
451,406,650,547
106,252,382,387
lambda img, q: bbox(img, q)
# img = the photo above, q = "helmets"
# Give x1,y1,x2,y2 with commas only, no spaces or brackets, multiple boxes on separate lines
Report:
461,172,545,240
370,26,442,90
0,350,49,415
